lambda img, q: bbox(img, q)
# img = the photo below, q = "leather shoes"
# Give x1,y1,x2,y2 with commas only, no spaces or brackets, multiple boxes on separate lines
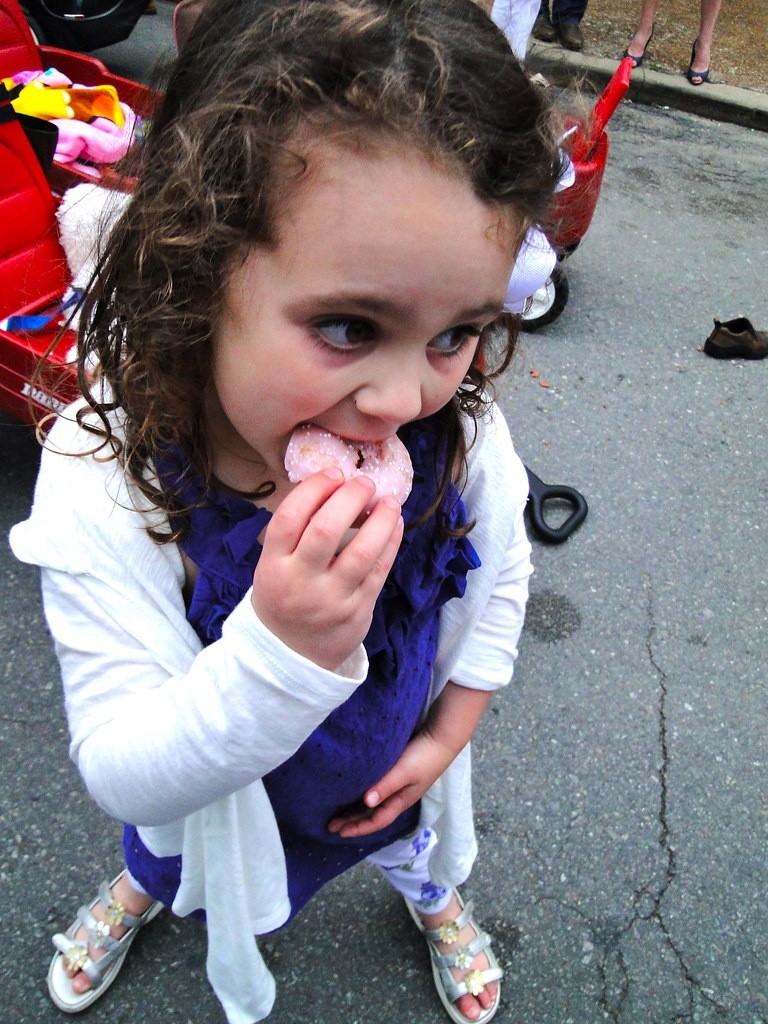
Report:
558,23,584,50
532,14,557,42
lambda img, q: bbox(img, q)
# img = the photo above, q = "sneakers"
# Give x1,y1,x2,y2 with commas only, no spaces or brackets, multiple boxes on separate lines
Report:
704,318,768,359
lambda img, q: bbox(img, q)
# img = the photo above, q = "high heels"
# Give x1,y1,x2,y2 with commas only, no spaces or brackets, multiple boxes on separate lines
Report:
623,29,653,68
688,39,709,85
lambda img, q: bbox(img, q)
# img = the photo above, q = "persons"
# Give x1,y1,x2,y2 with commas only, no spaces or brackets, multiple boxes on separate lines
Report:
623,0,722,86
7,0,588,1023
490,0,542,70
530,0,588,51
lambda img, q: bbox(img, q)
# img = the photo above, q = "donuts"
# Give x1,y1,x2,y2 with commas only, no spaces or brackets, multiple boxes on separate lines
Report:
283,424,413,513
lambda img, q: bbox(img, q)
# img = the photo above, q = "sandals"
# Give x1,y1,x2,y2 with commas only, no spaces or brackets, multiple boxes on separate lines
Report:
404,886,503,1024
47,867,165,1014
